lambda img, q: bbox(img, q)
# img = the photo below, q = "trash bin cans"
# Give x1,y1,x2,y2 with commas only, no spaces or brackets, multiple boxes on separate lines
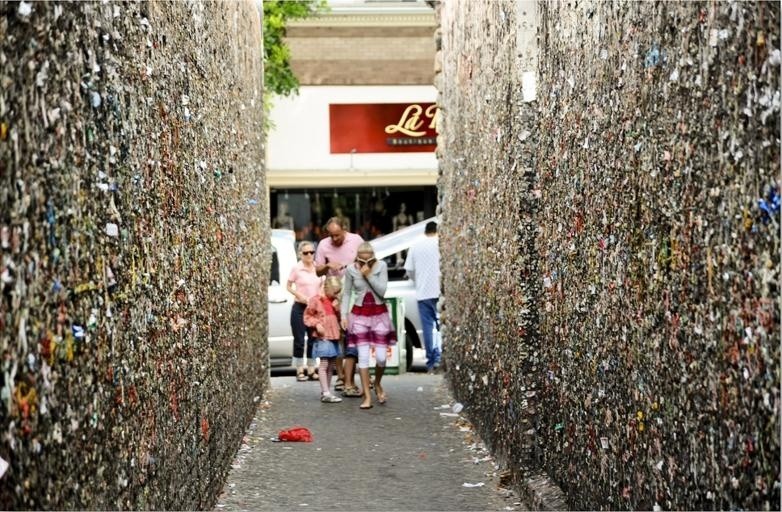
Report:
368,297,407,375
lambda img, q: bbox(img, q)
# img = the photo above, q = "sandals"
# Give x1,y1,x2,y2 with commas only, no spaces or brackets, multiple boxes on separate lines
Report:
321,378,388,406
296,372,319,381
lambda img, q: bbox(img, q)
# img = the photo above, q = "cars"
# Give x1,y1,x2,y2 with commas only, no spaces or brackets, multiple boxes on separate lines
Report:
268,229,308,372
367,216,440,371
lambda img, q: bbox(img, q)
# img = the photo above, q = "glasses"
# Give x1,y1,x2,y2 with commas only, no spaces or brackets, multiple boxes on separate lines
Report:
303,251,315,255
333,265,347,273
357,255,376,265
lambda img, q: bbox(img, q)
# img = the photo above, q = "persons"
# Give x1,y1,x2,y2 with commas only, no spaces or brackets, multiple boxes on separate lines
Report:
270,197,424,282
313,215,365,392
338,241,399,410
340,318,365,398
285,240,325,382
401,220,442,376
301,275,343,404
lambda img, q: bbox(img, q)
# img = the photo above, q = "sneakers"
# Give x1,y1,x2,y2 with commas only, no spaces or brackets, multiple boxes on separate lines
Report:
428,363,440,376
